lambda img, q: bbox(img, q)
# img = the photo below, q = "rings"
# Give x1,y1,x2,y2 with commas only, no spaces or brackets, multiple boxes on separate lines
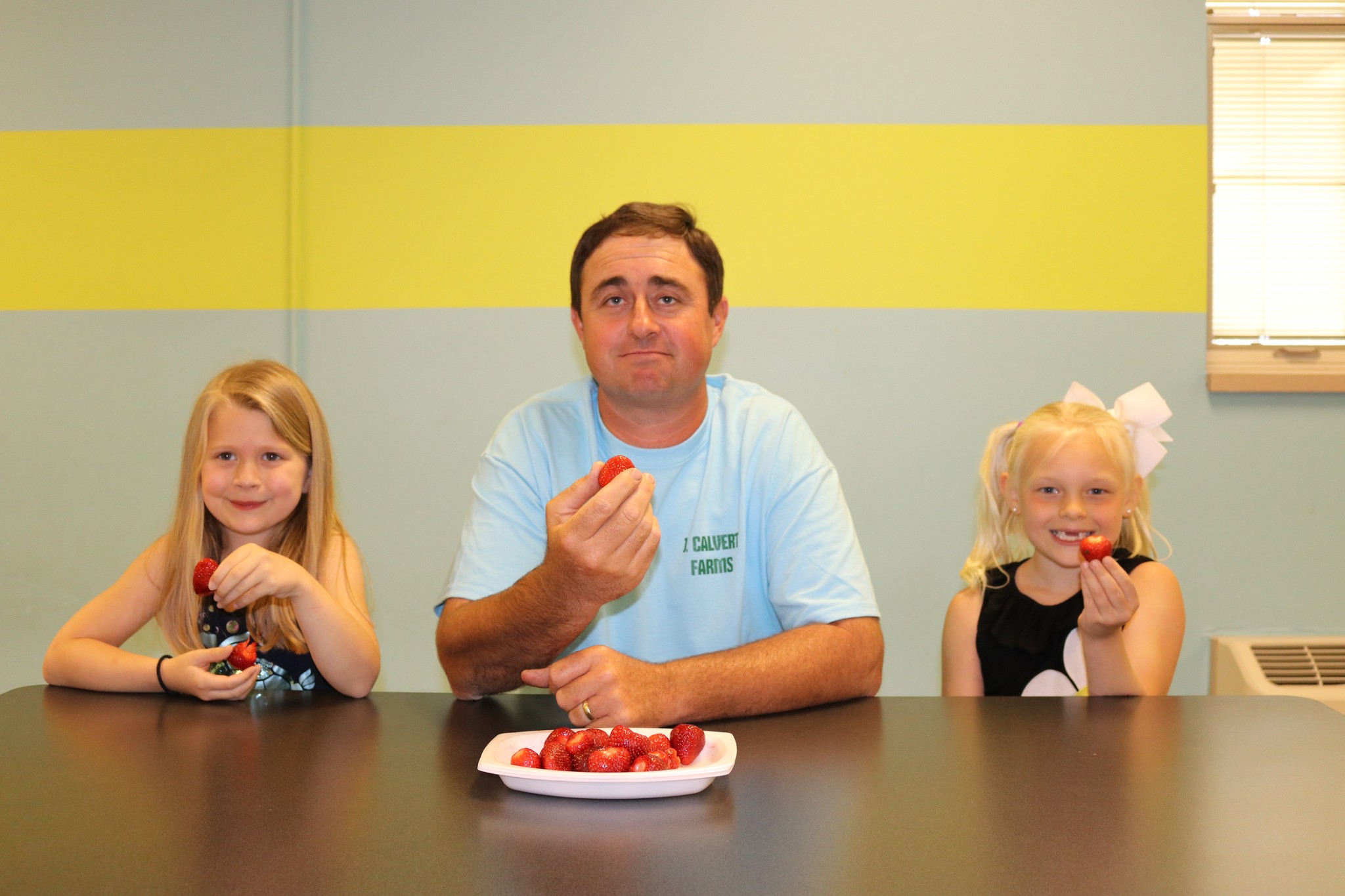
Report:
582,699,598,721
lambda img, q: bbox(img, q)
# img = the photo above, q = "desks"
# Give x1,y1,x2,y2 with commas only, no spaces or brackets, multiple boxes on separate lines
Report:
0,684,1344,896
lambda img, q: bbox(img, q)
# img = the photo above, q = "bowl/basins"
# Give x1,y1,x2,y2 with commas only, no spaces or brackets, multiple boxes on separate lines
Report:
477,727,739,798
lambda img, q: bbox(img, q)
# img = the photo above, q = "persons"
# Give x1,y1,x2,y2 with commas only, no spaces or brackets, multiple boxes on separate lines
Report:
42,358,382,701
433,203,884,726
941,380,1186,696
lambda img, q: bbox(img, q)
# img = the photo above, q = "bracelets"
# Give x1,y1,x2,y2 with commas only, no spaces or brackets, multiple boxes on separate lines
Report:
157,655,173,692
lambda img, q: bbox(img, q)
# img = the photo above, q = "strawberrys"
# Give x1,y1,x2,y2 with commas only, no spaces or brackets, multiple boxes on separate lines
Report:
192,558,221,597
228,637,257,671
539,725,680,772
598,455,635,488
510,747,542,769
1079,535,1113,564
670,723,705,765
